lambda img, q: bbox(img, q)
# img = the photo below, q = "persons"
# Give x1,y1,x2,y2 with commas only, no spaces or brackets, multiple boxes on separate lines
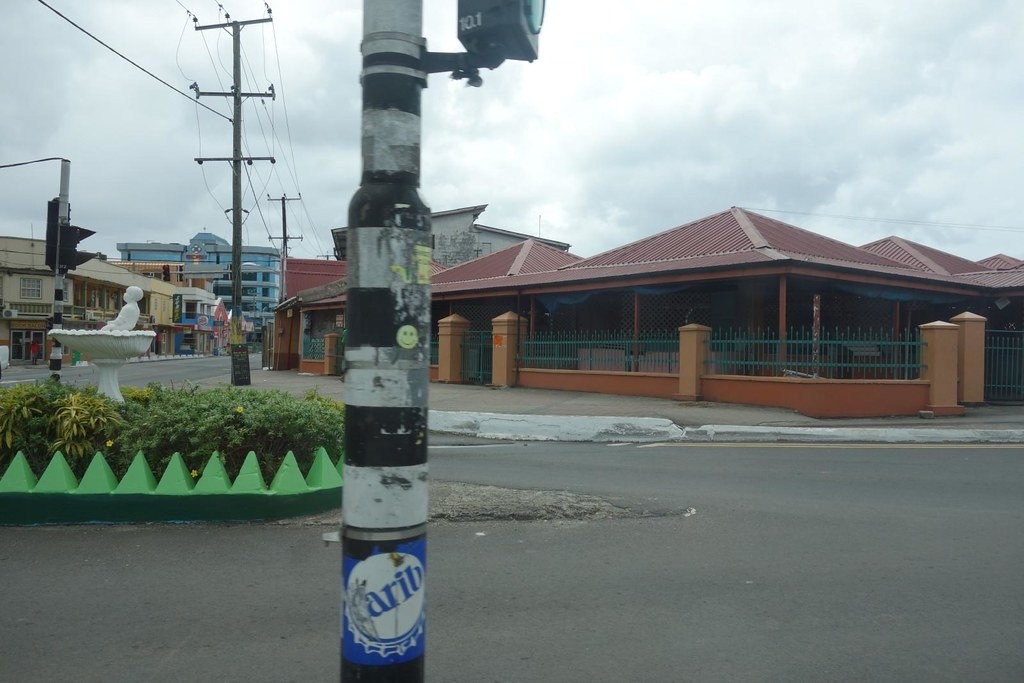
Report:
101,286,144,332
29,339,40,365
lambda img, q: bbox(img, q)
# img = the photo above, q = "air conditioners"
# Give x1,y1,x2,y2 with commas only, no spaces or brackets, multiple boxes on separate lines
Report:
2,308,18,318
0,296,5,308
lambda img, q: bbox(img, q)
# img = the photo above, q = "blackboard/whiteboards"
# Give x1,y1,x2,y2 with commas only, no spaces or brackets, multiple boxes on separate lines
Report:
231,344,251,386
